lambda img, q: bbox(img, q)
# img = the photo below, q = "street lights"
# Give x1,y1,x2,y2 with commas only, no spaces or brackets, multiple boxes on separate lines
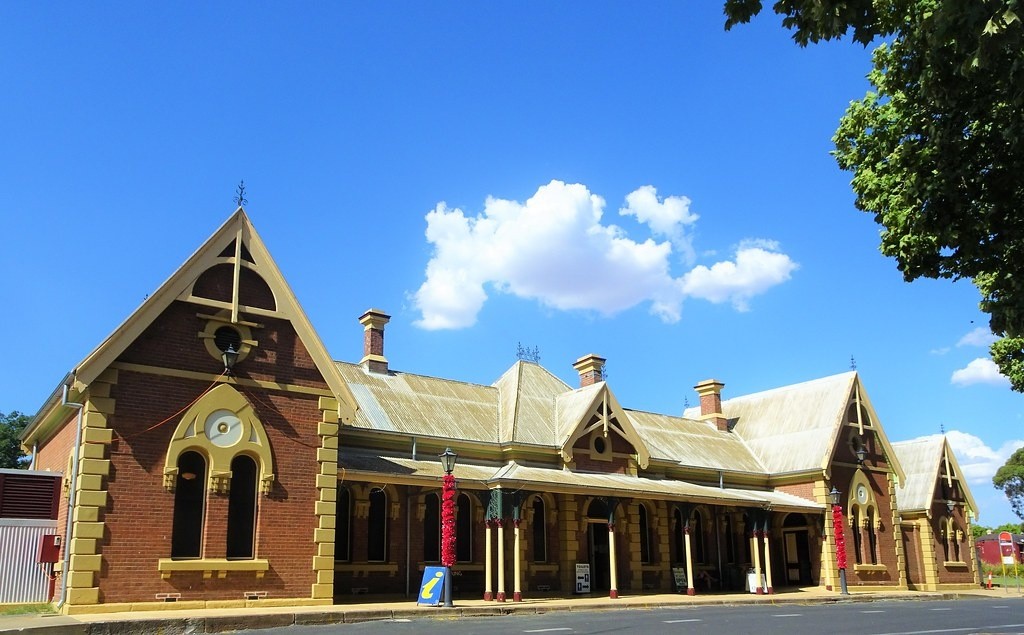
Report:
438,448,457,608
829,484,851,595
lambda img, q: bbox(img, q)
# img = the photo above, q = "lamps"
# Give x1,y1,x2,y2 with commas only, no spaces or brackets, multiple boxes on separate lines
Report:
221,343,240,380
857,447,867,464
946,501,955,514
